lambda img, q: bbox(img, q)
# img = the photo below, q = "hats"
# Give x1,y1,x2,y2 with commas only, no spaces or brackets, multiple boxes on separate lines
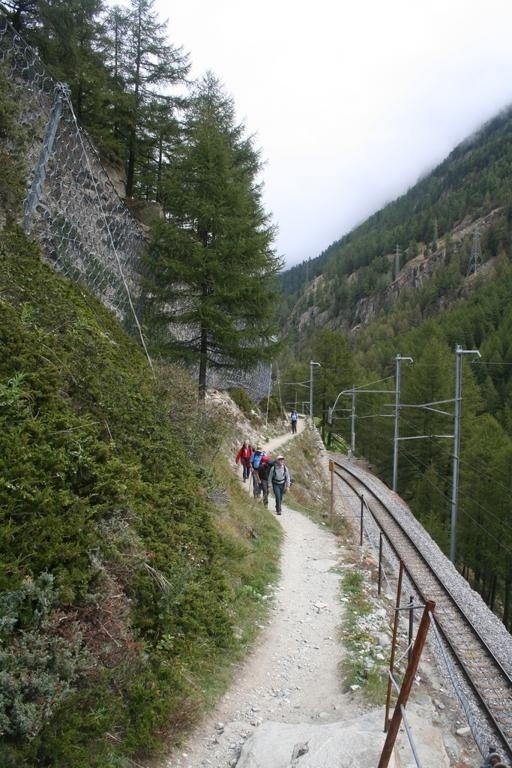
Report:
256,446,264,452
244,440,250,445
276,456,285,460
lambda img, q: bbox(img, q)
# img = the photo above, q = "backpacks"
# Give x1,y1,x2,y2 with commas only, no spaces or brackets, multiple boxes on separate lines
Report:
262,460,274,481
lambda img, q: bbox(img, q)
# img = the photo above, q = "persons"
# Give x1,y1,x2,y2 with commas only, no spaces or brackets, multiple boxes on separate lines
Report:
290,410,298,433
249,442,267,498
256,454,273,508
268,454,291,515
235,439,253,482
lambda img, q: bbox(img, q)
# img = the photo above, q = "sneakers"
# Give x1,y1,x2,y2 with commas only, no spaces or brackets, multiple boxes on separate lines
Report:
264,504,281,515
